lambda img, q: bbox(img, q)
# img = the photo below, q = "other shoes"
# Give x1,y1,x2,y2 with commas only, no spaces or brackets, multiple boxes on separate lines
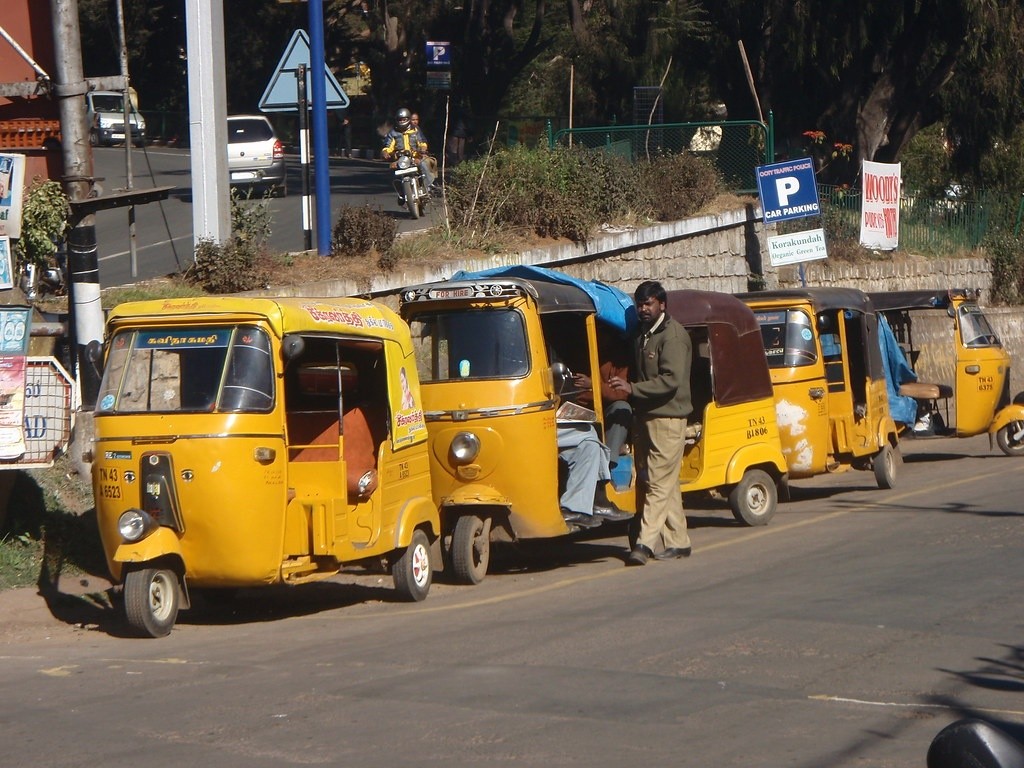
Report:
398,195,405,206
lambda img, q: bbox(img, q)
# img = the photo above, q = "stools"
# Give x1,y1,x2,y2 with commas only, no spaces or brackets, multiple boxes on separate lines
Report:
900,383,952,437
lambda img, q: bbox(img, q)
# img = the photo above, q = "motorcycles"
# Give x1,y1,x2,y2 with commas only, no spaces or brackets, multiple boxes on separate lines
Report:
91,295,444,642
355,275,646,590
612,289,793,543
734,285,905,497
387,146,439,219
863,289,1024,456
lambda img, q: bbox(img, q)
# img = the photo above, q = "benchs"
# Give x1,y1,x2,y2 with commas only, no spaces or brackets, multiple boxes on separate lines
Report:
286,357,386,500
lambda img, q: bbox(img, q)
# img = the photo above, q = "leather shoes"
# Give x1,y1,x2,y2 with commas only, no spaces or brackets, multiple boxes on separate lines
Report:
628,544,652,565
561,508,603,528
593,502,634,523
654,547,691,561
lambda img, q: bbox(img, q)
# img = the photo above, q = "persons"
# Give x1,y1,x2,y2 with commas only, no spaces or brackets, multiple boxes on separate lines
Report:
401,367,415,409
514,317,630,527
382,108,438,206
608,281,692,566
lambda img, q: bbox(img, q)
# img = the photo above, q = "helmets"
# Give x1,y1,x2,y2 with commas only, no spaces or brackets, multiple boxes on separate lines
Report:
393,108,411,131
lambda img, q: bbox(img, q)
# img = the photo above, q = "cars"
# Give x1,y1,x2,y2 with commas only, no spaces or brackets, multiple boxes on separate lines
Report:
84,91,147,148
226,115,288,198
